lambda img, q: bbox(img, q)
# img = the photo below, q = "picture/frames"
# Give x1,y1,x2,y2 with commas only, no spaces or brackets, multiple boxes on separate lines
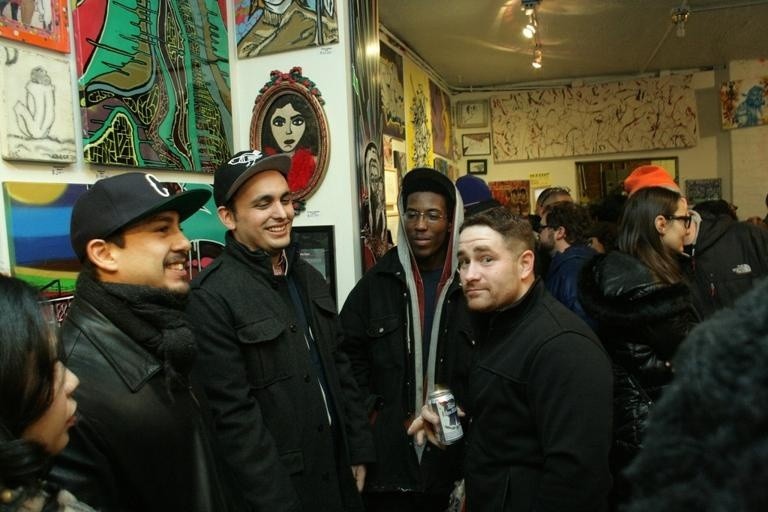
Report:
384,168,398,206
290,225,337,315
387,212,399,245
250,80,330,203
0,0,71,54
462,133,491,156
530,185,550,215
457,100,488,129
467,159,487,174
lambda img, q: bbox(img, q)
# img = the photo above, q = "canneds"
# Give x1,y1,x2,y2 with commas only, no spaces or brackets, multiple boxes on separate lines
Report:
427,389,464,446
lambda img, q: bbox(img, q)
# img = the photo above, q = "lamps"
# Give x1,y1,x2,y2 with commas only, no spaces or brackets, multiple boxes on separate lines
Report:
670,7,690,38
521,0,544,69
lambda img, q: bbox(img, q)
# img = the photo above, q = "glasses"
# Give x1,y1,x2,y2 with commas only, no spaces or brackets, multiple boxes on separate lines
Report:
663,214,692,230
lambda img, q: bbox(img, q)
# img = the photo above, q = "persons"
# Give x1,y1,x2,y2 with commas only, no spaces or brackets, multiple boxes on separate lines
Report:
406,205,615,511
187,149,374,510
338,167,466,511
450,164,768,511
0,272,98,511
59,171,230,511
260,94,320,195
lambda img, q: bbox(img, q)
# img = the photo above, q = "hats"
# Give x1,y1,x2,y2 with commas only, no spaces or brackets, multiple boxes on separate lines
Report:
623,163,684,199
213,149,292,209
69,172,212,268
455,173,492,209
402,167,457,210
